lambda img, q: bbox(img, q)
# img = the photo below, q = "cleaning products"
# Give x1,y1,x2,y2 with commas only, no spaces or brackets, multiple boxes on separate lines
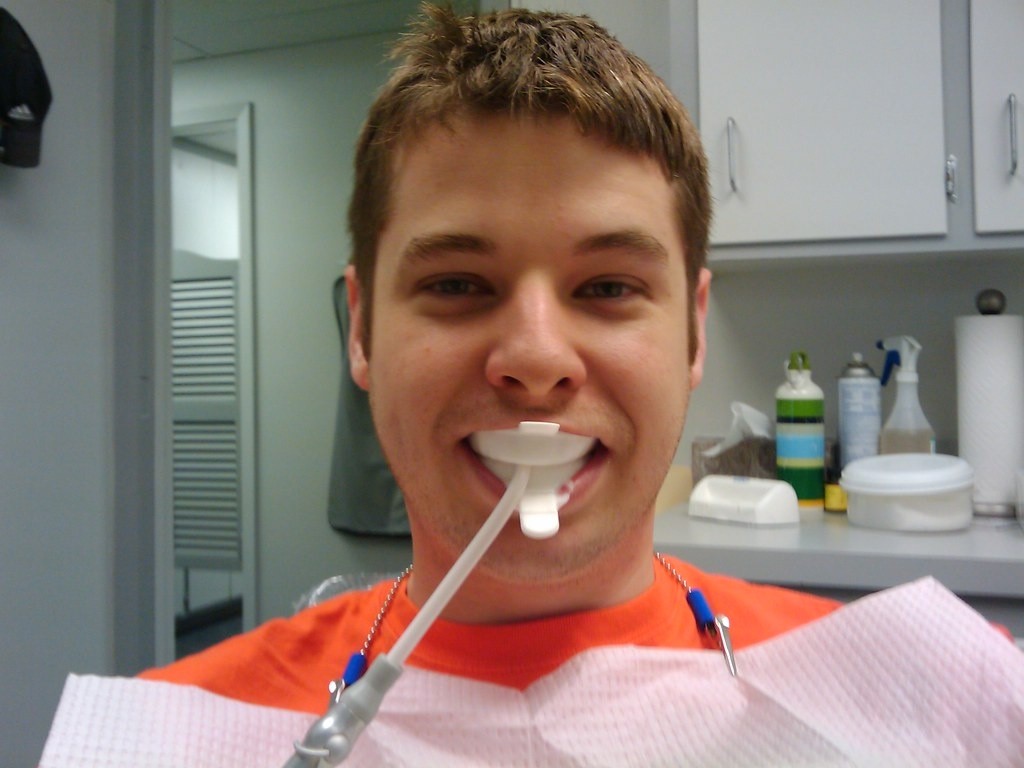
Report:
773,330,940,511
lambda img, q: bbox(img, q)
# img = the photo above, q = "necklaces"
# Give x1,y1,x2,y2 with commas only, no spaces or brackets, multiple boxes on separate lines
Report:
338,553,717,690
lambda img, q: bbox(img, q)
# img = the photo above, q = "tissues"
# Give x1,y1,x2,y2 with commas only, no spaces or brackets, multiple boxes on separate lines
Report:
684,401,778,497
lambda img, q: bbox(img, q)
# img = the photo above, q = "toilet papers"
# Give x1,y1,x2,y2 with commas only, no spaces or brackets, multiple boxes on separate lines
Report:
953,312,1023,513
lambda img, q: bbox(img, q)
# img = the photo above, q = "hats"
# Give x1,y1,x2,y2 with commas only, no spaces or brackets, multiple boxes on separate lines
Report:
0,7,52,168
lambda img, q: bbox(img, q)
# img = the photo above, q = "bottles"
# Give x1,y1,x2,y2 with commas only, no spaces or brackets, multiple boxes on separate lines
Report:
776,351,826,499
835,353,882,473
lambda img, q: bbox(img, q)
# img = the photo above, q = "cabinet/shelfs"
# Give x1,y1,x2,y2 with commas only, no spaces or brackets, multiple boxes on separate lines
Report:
669,0,1024,274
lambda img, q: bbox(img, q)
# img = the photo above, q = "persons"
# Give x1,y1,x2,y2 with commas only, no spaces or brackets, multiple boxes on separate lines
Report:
131,1,1024,767
